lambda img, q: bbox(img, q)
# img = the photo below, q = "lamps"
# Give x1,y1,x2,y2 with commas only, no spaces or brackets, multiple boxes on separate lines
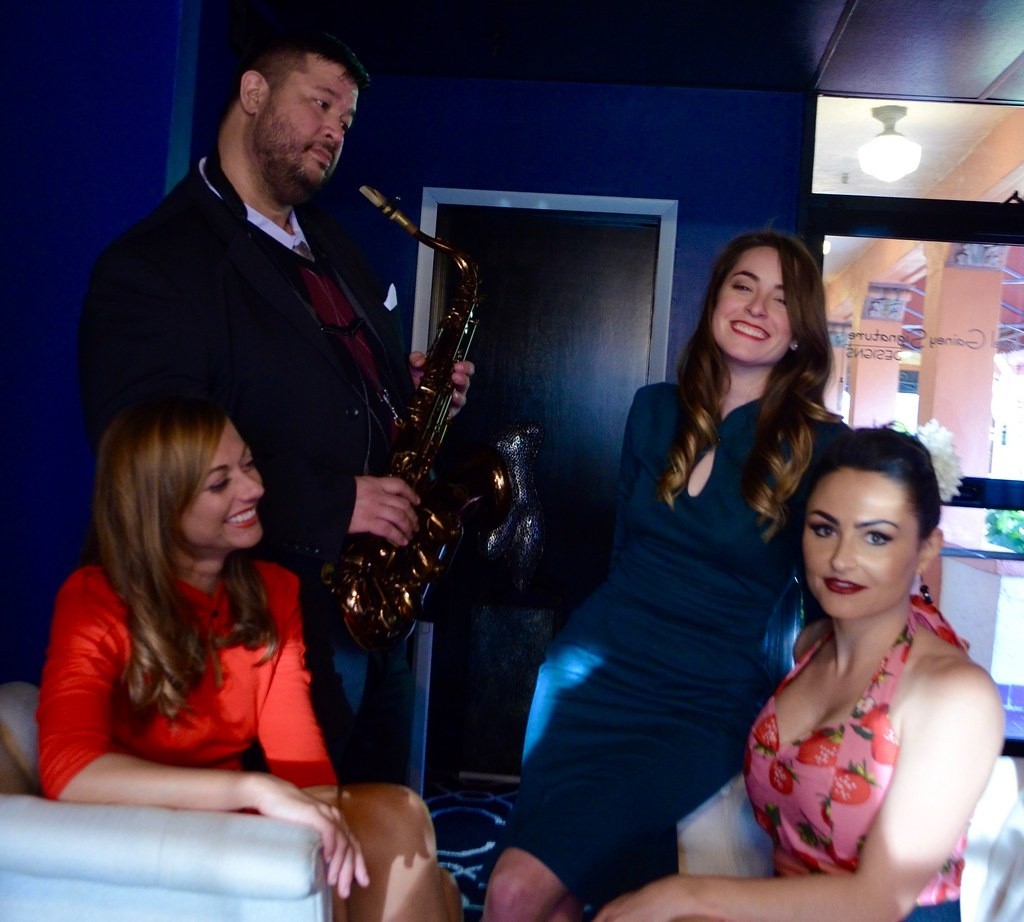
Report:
857,105,921,182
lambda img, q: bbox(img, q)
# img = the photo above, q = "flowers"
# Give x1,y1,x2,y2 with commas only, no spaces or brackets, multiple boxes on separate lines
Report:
916,420,963,503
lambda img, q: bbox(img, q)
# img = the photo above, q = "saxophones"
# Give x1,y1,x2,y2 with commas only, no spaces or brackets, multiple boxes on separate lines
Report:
326,183,517,650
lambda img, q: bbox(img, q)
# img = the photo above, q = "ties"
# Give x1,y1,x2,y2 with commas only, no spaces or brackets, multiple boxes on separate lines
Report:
292,240,314,262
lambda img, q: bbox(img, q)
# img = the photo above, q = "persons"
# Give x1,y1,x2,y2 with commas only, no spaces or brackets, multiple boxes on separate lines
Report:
35,397,464,922
486,234,942,922
595,428,1007,922
76,24,475,802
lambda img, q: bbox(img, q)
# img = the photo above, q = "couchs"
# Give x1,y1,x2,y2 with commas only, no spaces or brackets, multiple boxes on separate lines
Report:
675,738,1024,922
0,679,331,922
